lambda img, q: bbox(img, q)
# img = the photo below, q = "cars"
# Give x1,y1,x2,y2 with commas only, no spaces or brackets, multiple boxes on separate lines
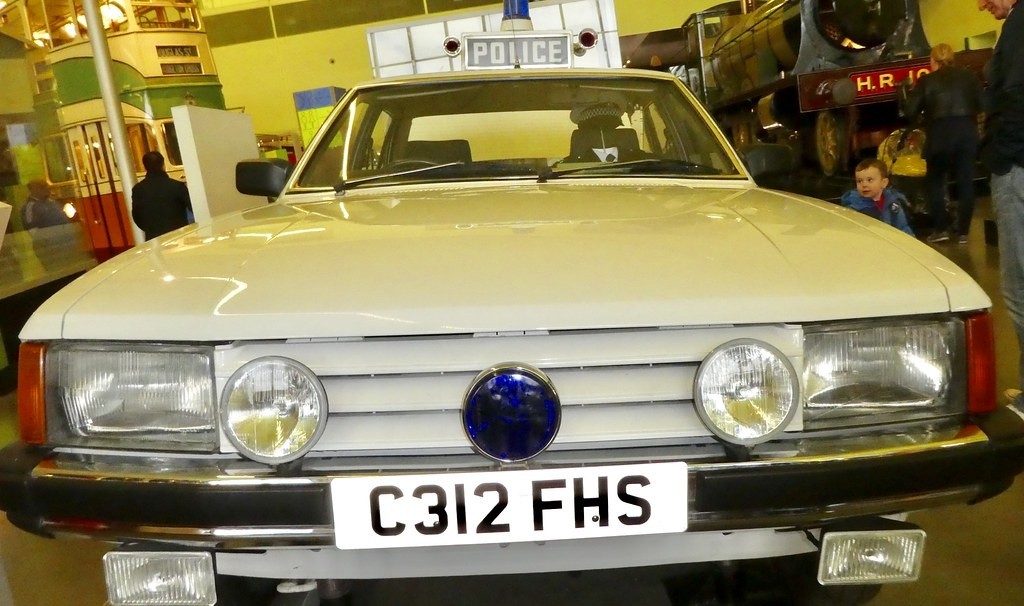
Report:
0,66,1024,606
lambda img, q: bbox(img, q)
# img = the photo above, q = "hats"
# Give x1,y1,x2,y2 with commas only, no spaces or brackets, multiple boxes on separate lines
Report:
27,179,53,191
570,92,627,127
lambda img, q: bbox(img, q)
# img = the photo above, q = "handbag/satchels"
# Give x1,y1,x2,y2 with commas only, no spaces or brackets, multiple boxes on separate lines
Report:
892,141,927,177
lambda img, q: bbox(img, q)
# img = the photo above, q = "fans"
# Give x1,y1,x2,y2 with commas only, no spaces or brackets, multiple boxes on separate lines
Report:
569,93,629,125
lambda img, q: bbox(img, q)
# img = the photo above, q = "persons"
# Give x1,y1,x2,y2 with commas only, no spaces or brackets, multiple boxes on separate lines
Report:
21,179,68,265
977,0,1024,407
841,160,916,238
898,43,983,244
556,93,652,163
131,150,194,241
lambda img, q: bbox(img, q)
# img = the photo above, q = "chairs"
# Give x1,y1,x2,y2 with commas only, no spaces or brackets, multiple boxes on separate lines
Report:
570,128,639,157
392,139,472,167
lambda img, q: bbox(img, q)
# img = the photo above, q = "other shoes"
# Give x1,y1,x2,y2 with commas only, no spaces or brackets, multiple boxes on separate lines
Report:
951,234,968,244
1004,389,1024,402
926,231,949,242
